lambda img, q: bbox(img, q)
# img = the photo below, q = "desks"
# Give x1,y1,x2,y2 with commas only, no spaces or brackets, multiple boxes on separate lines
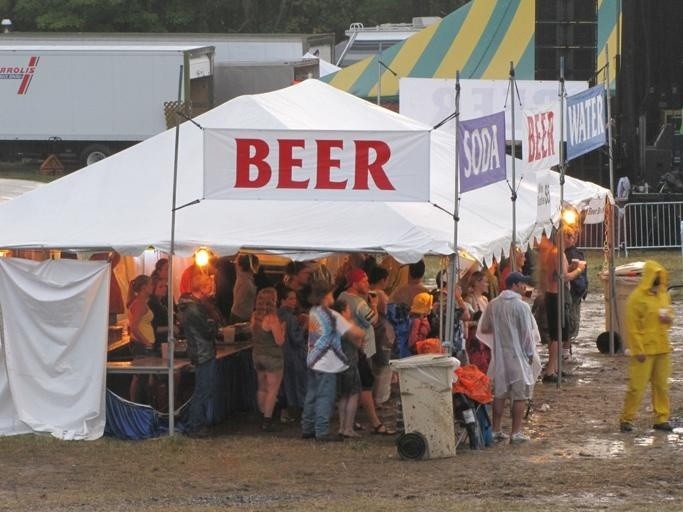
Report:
99,308,255,435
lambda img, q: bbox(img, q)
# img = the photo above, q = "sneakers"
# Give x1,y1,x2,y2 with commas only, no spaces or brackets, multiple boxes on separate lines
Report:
621,418,633,433
652,420,674,431
510,431,532,443
542,352,584,382
257,409,353,440
492,430,510,441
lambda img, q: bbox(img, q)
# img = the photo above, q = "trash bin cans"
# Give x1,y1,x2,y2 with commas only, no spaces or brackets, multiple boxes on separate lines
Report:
596,261,658,354
388,353,461,460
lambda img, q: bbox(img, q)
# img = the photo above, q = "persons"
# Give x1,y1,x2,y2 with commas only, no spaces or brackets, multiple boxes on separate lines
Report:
625,259,673,432
62,226,582,443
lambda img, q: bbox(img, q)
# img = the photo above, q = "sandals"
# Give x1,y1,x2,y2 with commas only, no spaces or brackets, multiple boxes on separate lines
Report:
371,423,400,437
354,421,368,433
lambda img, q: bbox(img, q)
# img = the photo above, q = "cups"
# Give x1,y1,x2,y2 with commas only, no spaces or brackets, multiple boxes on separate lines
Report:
222,327,236,342
162,343,176,360
660,309,670,317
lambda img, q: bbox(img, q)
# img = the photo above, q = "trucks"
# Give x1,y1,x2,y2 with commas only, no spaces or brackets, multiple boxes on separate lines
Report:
212,59,319,107
0,43,215,169
0,31,335,69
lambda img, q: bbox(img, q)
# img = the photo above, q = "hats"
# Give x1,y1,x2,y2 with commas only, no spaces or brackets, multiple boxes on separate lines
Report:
307,280,340,305
506,272,533,284
345,268,368,287
410,292,434,316
